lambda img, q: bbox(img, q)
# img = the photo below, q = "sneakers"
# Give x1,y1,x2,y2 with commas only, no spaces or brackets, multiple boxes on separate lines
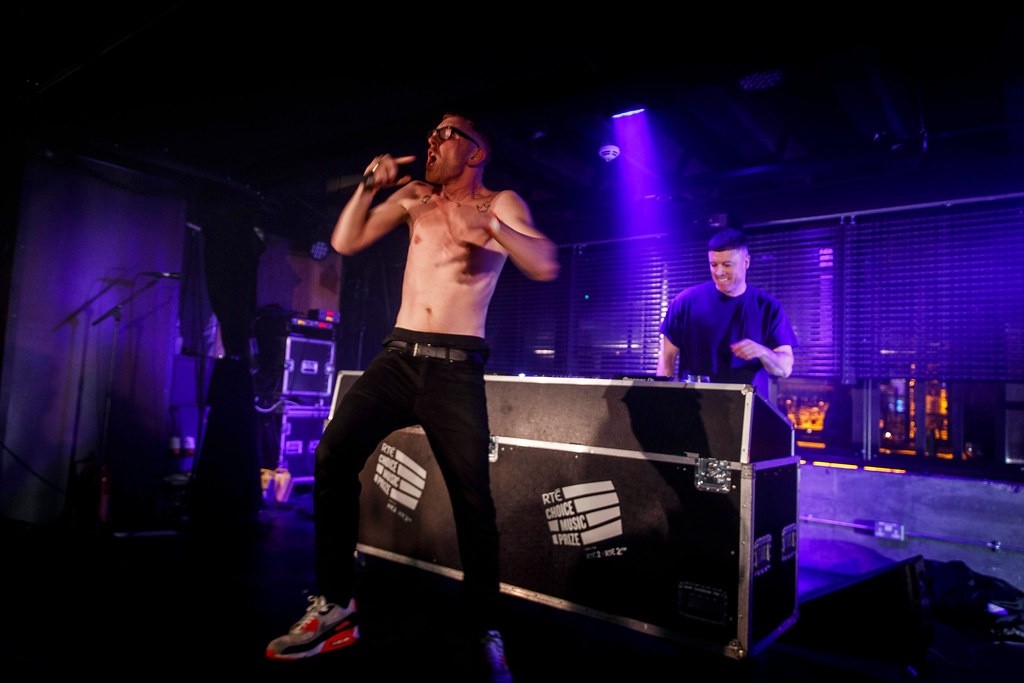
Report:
479,628,513,683
265,597,365,660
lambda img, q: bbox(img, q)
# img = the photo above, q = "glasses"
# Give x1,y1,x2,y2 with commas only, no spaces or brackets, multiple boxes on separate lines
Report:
427,126,480,150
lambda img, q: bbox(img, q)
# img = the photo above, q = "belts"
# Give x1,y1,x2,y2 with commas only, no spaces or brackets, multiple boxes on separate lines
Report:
387,340,479,363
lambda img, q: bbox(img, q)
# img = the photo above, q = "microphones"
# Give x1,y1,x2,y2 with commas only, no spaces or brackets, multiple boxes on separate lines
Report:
143,271,180,279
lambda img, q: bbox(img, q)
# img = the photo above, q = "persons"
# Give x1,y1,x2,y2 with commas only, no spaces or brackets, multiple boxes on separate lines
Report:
266,111,560,683
656,227,802,385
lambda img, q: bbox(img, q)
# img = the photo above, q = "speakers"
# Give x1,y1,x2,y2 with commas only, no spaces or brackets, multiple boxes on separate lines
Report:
797,555,935,667
